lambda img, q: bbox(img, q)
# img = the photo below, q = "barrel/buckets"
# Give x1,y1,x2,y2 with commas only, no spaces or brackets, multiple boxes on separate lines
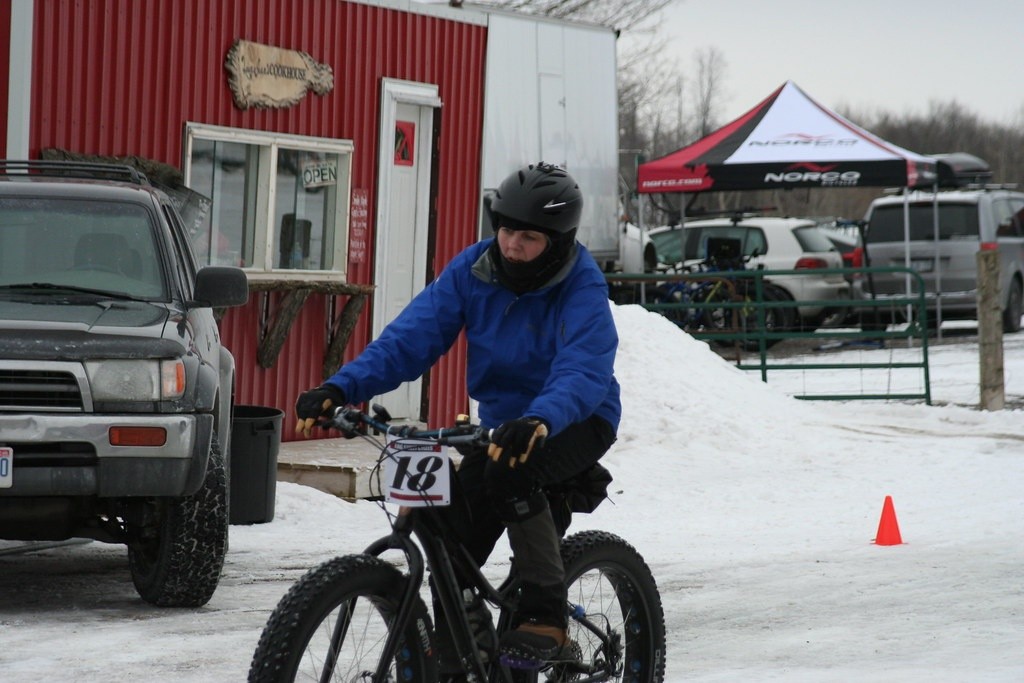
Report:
227,405,286,526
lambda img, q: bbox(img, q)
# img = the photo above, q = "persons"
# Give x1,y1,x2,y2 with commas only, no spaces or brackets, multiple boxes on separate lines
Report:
296,162,621,683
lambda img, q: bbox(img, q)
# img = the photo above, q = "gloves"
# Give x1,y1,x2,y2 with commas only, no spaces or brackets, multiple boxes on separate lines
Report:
484,416,552,466
294,384,340,435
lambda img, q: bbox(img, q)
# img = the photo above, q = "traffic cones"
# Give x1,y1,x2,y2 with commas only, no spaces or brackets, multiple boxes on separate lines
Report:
872,495,906,545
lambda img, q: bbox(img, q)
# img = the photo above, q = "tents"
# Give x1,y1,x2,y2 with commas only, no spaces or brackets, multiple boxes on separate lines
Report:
636,79,953,349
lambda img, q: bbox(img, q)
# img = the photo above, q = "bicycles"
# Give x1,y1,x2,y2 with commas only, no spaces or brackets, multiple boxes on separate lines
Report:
608,261,744,350
248,401,666,683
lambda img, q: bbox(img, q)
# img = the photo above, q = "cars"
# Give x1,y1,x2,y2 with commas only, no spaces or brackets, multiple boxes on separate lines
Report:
822,228,864,291
648,215,847,354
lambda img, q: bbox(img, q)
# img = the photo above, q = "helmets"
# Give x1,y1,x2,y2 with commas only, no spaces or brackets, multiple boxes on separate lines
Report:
484,162,588,290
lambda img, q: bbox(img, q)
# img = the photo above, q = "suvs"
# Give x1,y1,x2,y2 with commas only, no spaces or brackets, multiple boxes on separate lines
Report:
854,185,1023,335
0,162,252,610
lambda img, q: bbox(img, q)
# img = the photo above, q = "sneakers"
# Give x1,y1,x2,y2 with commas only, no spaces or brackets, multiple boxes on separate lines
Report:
502,620,574,657
